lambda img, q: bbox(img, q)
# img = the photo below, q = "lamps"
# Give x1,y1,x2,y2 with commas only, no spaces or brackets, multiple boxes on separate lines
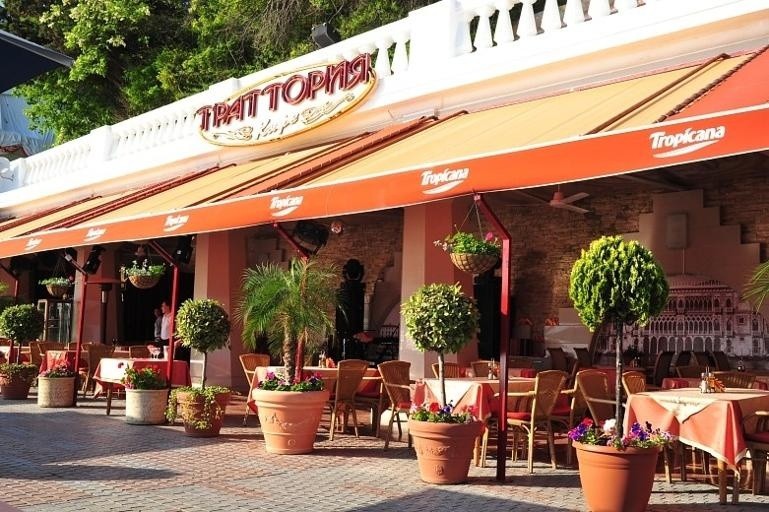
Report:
329,217,343,234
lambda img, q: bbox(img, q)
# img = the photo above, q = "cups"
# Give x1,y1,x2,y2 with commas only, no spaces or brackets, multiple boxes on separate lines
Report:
163,346,170,361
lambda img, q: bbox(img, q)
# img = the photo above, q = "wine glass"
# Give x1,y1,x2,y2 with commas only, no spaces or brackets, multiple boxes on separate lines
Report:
148,347,161,360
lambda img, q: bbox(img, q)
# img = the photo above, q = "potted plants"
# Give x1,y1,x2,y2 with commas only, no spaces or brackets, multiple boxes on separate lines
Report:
232,251,340,459
115,361,173,430
38,250,74,298
161,297,232,443
433,193,502,275
400,280,488,486
119,240,169,290
564,232,671,512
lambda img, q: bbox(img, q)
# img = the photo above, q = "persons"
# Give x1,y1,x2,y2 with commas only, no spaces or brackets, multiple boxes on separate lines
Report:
153,308,162,342
159,301,173,346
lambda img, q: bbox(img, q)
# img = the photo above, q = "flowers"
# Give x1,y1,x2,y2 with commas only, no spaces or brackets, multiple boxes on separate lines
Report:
505,183,591,215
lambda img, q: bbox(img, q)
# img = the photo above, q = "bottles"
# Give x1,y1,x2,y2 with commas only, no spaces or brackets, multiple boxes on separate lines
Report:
459,366,477,380
318,349,336,368
110,335,121,346
698,366,717,394
488,358,500,380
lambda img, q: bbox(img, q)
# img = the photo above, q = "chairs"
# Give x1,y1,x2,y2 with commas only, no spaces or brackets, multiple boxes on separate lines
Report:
1,337,188,417
235,345,769,506
